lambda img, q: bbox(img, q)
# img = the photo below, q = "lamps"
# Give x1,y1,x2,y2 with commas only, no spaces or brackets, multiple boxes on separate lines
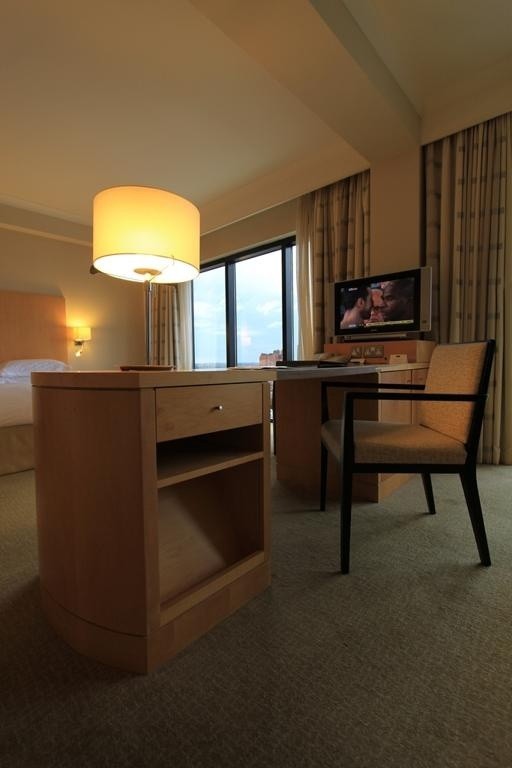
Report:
87,183,204,372
73,326,93,357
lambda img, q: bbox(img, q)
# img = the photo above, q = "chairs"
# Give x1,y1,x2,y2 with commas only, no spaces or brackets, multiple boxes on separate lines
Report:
314,337,498,578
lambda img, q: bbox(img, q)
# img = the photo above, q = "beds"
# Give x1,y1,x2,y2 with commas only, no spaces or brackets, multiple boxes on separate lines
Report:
0,289,70,476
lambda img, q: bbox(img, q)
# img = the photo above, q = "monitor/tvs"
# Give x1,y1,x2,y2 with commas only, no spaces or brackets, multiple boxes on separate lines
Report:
327,266,432,342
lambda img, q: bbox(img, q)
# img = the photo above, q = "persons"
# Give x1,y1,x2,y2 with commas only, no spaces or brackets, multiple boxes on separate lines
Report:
341,278,376,332
379,280,413,324
362,288,385,324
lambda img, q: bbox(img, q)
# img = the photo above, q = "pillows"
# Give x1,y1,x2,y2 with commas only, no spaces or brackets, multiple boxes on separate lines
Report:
1,358,63,384
